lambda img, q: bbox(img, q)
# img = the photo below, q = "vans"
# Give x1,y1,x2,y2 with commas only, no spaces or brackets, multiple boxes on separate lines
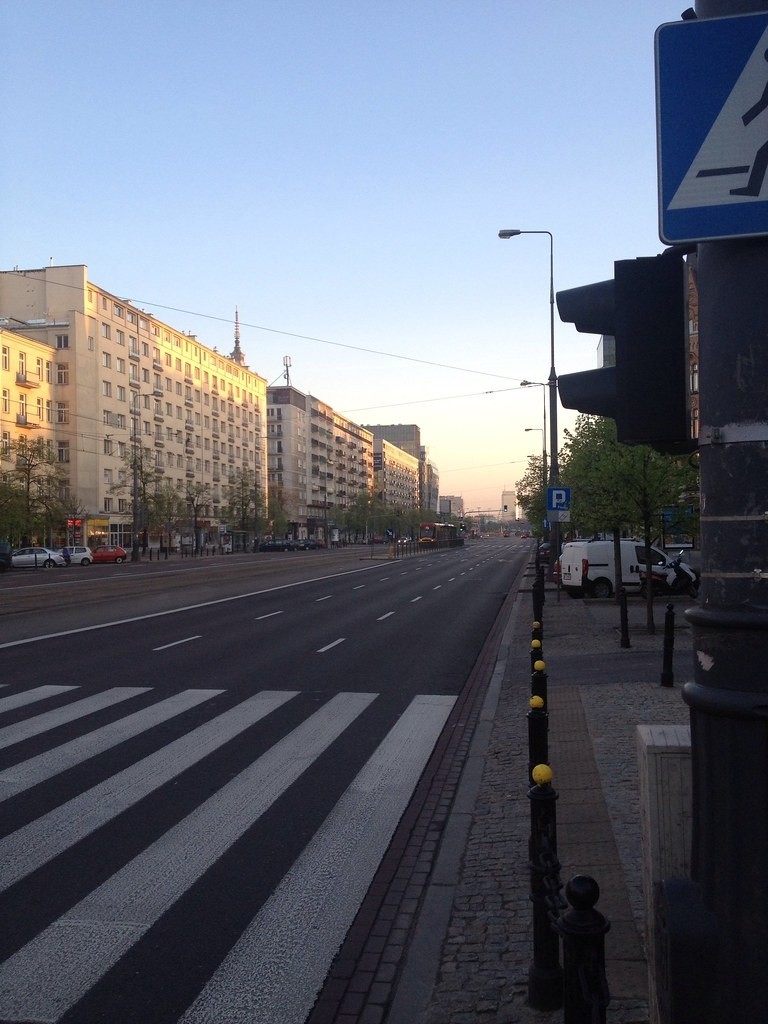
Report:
561,542,695,599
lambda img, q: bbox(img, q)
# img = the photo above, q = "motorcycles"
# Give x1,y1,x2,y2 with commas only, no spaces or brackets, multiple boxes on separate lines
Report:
638,548,696,604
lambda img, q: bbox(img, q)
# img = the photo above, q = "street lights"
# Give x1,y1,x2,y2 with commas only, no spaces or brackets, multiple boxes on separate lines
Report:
498,228,558,590
129,391,165,564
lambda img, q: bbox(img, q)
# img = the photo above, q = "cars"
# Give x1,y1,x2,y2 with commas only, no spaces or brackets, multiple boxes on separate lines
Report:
397,537,409,544
90,545,127,564
58,546,94,566
11,547,65,570
503,529,529,539
259,538,325,552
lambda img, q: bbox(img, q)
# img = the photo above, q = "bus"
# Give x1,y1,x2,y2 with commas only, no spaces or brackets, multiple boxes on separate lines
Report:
418,522,457,546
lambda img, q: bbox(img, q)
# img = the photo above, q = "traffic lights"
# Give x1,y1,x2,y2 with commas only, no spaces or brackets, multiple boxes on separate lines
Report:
503,505,508,512
